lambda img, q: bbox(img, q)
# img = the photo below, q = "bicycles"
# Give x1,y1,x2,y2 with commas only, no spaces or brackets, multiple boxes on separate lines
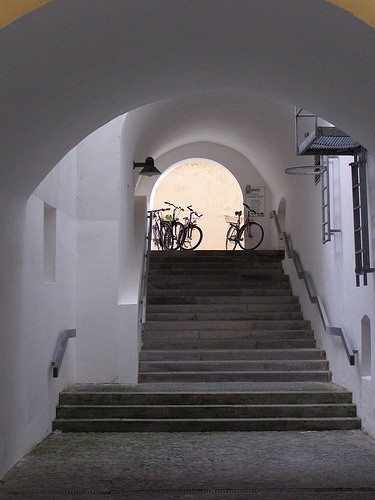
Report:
226,203,264,251
176,205,203,251
161,202,186,250
151,208,175,252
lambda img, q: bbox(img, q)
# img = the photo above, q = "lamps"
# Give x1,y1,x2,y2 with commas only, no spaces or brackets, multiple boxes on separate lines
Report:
132,157,162,177
